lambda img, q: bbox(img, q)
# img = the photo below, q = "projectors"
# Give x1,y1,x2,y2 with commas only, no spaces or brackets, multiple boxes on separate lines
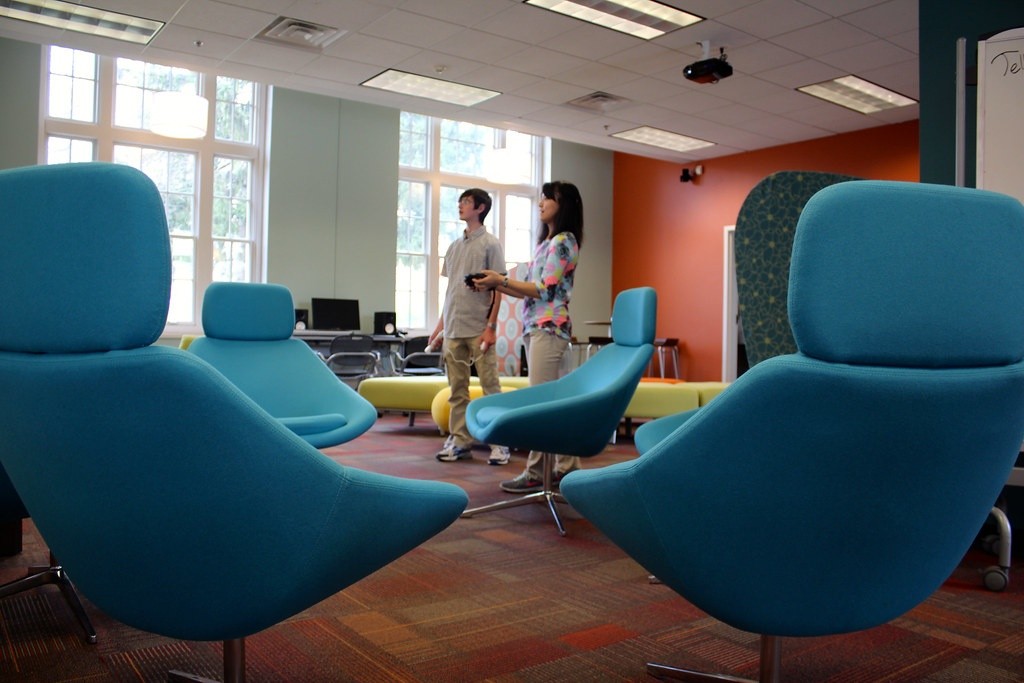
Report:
683,58,733,83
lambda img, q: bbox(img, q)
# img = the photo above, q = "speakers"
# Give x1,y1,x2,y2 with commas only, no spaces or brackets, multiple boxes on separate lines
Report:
295,309,308,329
374,312,396,336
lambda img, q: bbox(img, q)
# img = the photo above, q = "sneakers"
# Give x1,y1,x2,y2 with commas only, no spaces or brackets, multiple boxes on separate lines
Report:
436,444,472,462
488,447,510,464
500,474,560,491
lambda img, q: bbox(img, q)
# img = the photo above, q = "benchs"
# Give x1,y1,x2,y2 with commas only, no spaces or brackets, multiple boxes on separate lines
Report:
678,382,730,407
358,375,699,441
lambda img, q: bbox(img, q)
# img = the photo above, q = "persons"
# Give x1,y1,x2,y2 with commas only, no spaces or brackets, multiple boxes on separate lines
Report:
464,180,584,492
428,189,510,465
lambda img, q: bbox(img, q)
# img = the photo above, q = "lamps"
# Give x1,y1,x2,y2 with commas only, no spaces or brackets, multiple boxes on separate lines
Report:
680,168,694,183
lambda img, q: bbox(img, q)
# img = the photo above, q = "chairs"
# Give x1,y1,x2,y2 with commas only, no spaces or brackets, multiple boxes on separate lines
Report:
184,282,378,451
560,179,1023,683
453,287,658,540
1,159,467,683
318,330,446,393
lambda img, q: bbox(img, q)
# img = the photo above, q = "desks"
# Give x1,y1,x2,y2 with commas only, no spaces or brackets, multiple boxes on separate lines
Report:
584,320,611,337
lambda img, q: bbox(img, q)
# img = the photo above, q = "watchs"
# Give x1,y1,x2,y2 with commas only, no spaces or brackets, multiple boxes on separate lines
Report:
487,322,497,331
504,276,509,288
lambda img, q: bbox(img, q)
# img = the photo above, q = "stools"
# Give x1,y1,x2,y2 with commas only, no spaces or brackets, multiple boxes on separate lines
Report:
431,386,519,437
558,337,681,378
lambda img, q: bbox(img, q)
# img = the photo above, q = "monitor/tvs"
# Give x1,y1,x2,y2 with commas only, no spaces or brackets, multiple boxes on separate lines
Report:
311,297,360,332
